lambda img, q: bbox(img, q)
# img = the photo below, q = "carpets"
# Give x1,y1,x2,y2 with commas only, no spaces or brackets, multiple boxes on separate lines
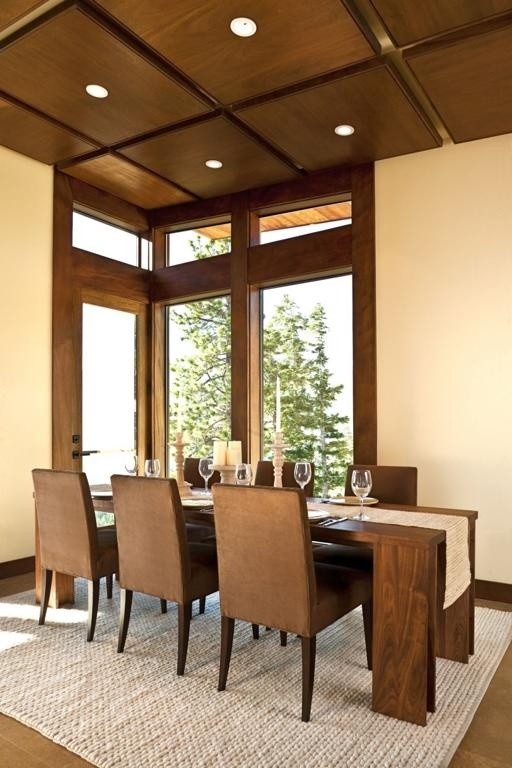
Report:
1,572,511,768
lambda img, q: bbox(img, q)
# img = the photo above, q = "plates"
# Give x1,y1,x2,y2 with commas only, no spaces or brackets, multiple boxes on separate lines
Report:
307,510,330,520
329,495,380,506
180,500,214,510
90,491,113,501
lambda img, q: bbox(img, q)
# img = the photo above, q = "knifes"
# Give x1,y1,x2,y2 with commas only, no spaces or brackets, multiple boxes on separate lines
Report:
324,517,348,527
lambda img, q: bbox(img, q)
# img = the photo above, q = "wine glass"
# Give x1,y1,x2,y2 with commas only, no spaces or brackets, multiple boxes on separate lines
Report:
293,462,312,491
350,469,373,522
198,458,215,496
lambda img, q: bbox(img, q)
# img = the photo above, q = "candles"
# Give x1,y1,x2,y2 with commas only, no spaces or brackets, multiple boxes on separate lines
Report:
276,376,280,432
213,440,242,465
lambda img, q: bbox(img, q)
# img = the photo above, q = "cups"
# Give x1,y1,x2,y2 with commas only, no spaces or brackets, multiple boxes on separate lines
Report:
144,458,164,479
123,455,139,476
235,464,252,486
